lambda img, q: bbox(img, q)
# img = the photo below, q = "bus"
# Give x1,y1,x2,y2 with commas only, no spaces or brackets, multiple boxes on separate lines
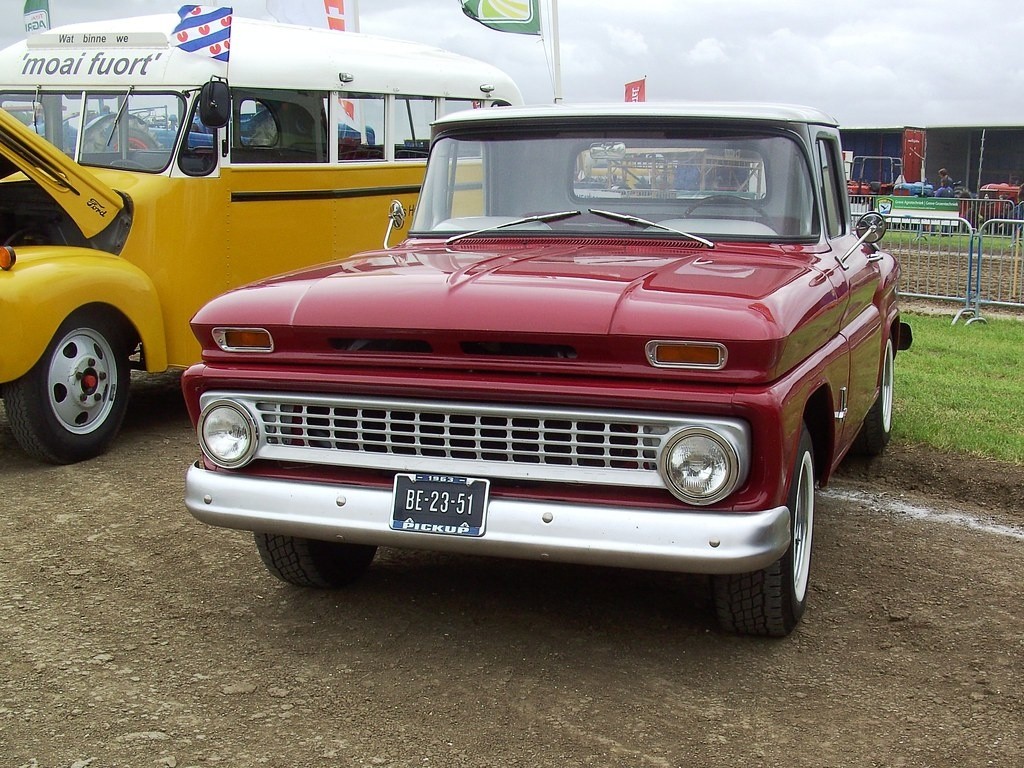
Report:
575,149,706,190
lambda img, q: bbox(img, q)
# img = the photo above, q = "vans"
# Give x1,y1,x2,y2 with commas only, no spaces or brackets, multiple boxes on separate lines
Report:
1,10,526,468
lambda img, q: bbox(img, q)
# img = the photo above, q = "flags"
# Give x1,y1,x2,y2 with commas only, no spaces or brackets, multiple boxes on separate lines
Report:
23,0,51,33
170,4,233,62
459,0,541,35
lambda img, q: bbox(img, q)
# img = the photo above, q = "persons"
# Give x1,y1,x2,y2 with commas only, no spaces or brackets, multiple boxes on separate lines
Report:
931,169,1024,237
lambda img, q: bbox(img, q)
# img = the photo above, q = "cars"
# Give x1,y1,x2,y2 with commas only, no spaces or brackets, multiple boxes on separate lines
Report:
178,100,915,640
